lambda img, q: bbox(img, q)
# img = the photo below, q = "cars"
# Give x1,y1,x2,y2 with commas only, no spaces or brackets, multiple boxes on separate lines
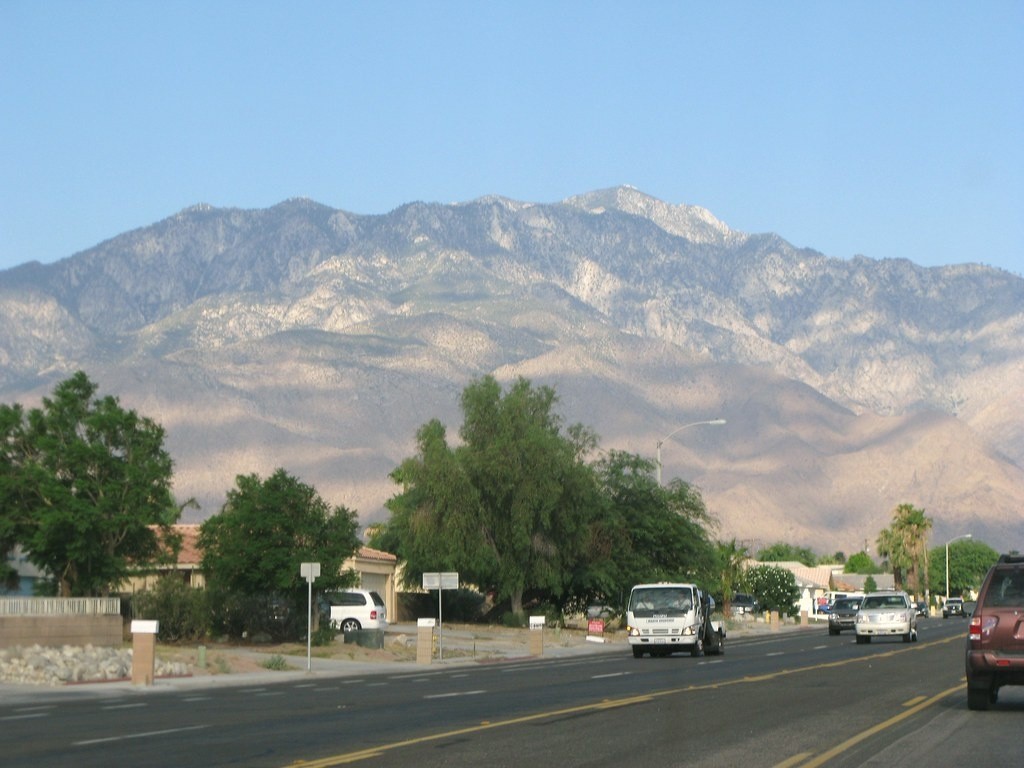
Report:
730,592,760,617
817,605,831,616
911,602,930,618
323,587,388,633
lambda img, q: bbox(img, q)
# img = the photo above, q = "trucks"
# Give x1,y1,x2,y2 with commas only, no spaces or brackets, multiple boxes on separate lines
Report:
626,582,728,657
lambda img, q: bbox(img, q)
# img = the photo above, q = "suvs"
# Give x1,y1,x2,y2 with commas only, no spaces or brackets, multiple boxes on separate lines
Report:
942,597,966,619
961,552,1024,710
826,596,864,635
852,588,919,642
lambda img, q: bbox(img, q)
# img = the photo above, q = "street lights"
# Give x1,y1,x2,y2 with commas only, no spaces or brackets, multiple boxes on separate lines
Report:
946,534,972,598
658,418,728,488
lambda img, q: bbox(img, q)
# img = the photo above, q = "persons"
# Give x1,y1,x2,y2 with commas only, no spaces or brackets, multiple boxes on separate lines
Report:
673,591,691,609
874,598,886,609
997,573,1024,606
637,594,654,610
